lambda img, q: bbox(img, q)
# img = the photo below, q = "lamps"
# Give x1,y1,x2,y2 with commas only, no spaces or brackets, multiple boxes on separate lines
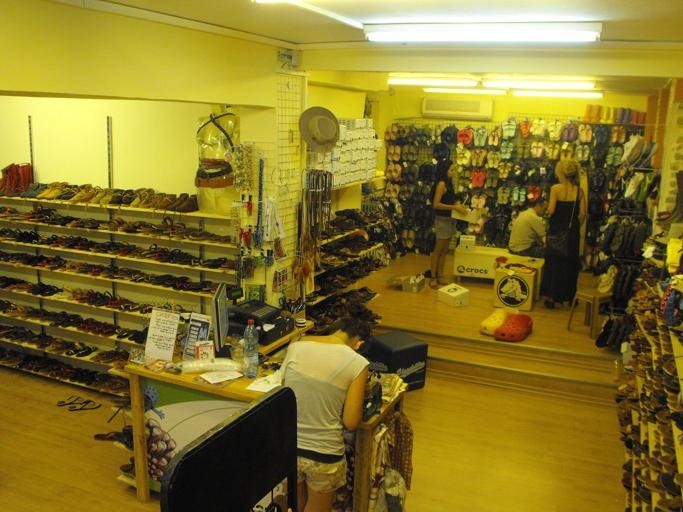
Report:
363,22,603,43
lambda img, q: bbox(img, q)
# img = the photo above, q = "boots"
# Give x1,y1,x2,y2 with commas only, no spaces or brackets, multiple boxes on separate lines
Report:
20,182,198,212
657,171,683,230
582,104,646,124
0,163,31,197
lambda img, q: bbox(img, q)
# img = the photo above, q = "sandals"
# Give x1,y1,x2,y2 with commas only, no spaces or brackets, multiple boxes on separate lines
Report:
429,277,448,290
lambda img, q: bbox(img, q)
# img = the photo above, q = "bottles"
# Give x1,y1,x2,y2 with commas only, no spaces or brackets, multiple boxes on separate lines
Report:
243,319,260,378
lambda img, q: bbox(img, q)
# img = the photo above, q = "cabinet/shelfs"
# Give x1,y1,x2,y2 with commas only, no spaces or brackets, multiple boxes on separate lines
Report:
309,173,388,298
383,115,683,461
1,194,237,399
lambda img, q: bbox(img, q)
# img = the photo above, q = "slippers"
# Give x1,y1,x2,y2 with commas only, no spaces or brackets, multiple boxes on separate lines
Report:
561,125,657,276
0,203,236,293
502,118,562,207
94,432,121,440
384,162,436,255
384,125,430,161
67,400,100,410
57,395,85,406
0,288,152,393
455,127,501,234
596,264,682,512
479,307,532,342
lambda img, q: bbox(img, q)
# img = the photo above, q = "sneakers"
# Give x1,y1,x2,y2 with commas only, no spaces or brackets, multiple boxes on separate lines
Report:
305,209,396,327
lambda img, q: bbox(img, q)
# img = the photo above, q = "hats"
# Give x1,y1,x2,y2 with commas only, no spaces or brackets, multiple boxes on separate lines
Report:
300,107,339,154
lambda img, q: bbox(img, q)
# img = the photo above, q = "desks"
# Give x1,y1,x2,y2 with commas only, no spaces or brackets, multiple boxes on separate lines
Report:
108,352,409,512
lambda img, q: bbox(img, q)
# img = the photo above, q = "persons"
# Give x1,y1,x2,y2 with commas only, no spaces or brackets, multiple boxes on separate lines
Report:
540,158,589,309
279,317,374,512
428,160,470,290
507,196,549,259
